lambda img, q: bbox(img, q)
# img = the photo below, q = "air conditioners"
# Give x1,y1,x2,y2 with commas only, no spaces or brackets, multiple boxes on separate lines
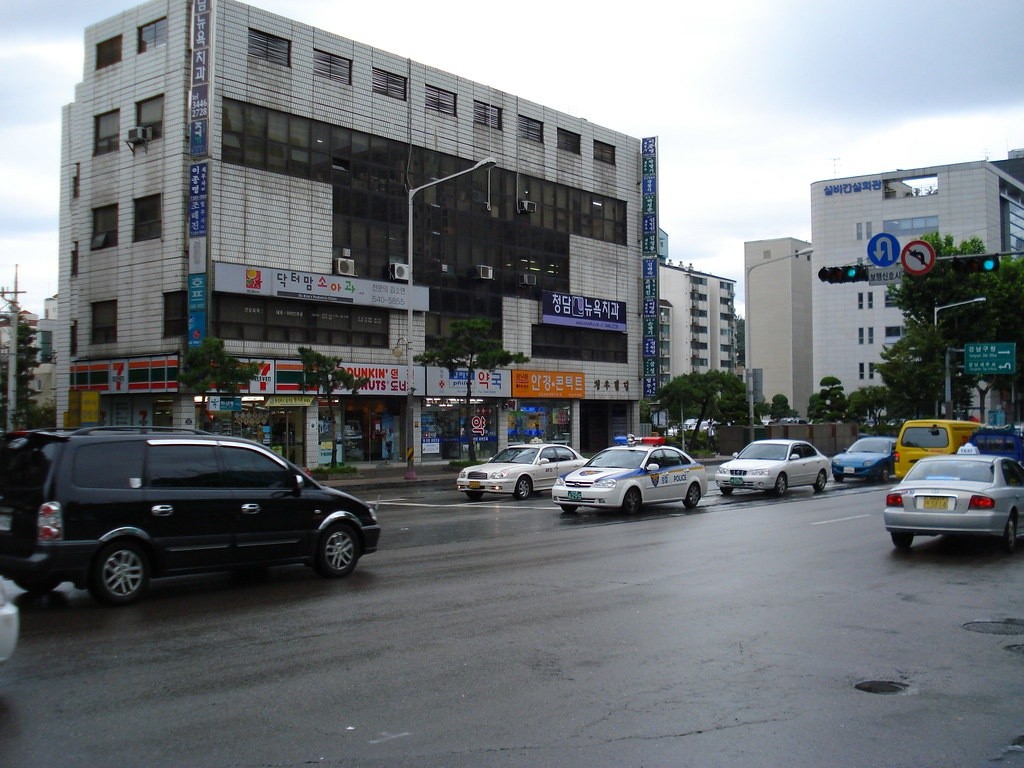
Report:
519,199,537,213
391,263,409,280
476,264,494,280
334,258,355,276
519,274,536,285
128,127,152,139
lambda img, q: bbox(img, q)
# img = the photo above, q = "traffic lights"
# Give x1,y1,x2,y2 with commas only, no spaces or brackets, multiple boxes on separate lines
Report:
965,256,999,273
817,266,869,283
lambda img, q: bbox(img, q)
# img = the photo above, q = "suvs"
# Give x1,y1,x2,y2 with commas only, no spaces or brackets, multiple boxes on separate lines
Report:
0,425,383,611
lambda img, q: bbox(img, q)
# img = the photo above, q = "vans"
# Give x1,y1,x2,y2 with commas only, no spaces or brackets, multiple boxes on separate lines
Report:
894,420,984,482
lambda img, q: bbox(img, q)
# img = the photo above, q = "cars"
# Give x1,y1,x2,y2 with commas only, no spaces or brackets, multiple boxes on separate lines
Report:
863,420,879,428
456,443,590,501
831,437,897,484
662,418,735,437
760,417,815,425
881,442,1024,556
551,434,709,518
887,419,897,426
714,438,832,496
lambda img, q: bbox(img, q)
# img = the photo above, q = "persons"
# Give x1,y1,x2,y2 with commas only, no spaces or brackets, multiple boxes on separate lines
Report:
707,421,718,454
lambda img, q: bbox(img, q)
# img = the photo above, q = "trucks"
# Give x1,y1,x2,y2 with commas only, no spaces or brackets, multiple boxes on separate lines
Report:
969,430,1024,481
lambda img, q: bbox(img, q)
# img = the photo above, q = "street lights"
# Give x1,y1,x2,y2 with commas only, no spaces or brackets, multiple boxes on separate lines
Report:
391,156,498,480
745,248,816,443
934,297,987,417
